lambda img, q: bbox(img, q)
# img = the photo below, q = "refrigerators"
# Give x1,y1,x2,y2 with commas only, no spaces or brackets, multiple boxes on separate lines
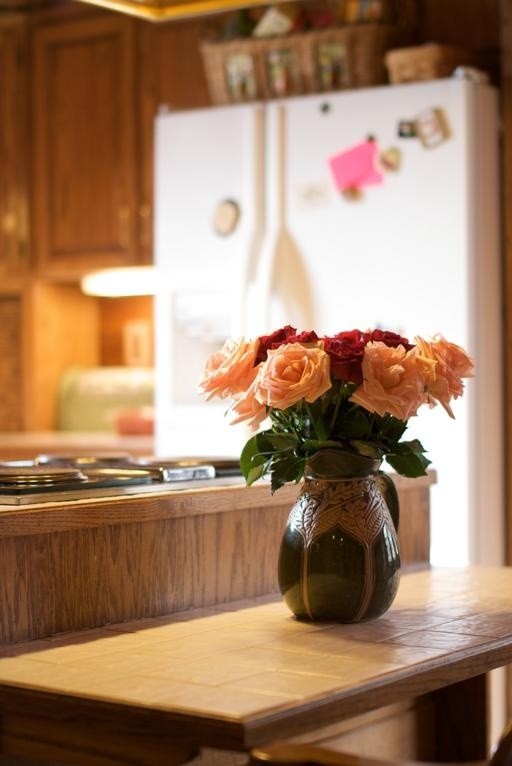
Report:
148,75,507,765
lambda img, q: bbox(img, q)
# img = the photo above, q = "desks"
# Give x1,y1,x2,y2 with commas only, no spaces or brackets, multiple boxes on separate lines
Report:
0,560,512,766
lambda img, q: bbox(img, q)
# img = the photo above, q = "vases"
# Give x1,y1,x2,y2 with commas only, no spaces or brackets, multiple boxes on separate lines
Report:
277,476,401,627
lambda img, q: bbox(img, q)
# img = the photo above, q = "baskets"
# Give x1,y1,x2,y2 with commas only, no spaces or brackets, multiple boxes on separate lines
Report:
384,40,468,84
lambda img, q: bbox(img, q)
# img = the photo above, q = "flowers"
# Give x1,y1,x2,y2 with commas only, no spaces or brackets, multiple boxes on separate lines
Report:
198,322,476,537
